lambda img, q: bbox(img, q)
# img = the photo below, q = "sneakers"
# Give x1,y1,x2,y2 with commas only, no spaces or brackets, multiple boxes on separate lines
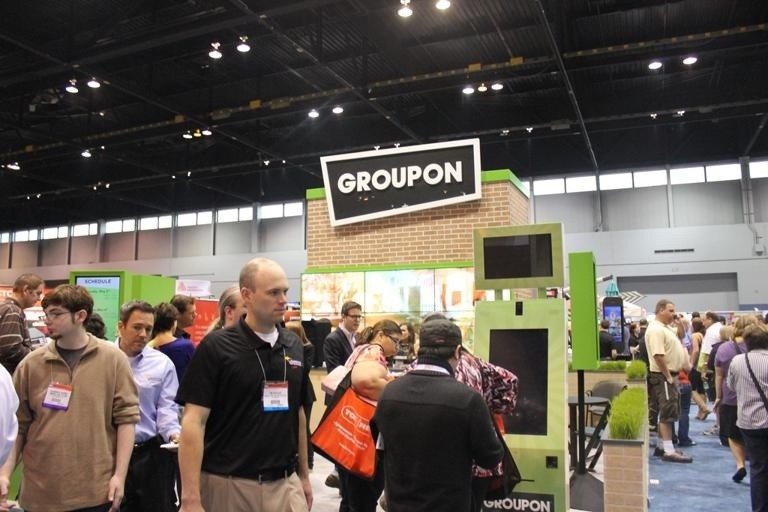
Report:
649,406,746,481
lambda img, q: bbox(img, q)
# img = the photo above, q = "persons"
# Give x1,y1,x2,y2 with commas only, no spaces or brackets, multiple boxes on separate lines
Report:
598,319,617,361
621,309,767,512
1,283,521,512
0,272,44,374
644,300,692,463
174,256,313,512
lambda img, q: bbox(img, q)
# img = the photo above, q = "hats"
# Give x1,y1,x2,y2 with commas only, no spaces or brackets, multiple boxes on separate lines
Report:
420,318,462,346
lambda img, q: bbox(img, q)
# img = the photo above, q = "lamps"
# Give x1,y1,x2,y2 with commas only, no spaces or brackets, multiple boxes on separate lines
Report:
0,0,702,156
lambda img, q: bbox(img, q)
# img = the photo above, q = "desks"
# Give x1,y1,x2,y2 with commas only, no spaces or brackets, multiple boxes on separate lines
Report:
567,394,610,476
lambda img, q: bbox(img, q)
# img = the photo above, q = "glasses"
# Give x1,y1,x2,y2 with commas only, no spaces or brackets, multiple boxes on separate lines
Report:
348,314,361,319
39,312,70,321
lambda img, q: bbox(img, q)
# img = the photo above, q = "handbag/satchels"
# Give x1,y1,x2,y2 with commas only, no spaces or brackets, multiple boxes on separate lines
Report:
477,417,521,500
321,364,380,406
310,372,380,485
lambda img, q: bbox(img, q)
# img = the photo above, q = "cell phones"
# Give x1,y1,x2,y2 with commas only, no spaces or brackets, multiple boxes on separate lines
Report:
602,297,625,353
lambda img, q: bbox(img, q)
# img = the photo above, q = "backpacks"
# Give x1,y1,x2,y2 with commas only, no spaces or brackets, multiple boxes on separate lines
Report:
478,362,519,414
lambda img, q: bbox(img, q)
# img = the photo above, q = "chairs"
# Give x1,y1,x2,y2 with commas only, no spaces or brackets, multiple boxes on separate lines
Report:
582,379,625,447
